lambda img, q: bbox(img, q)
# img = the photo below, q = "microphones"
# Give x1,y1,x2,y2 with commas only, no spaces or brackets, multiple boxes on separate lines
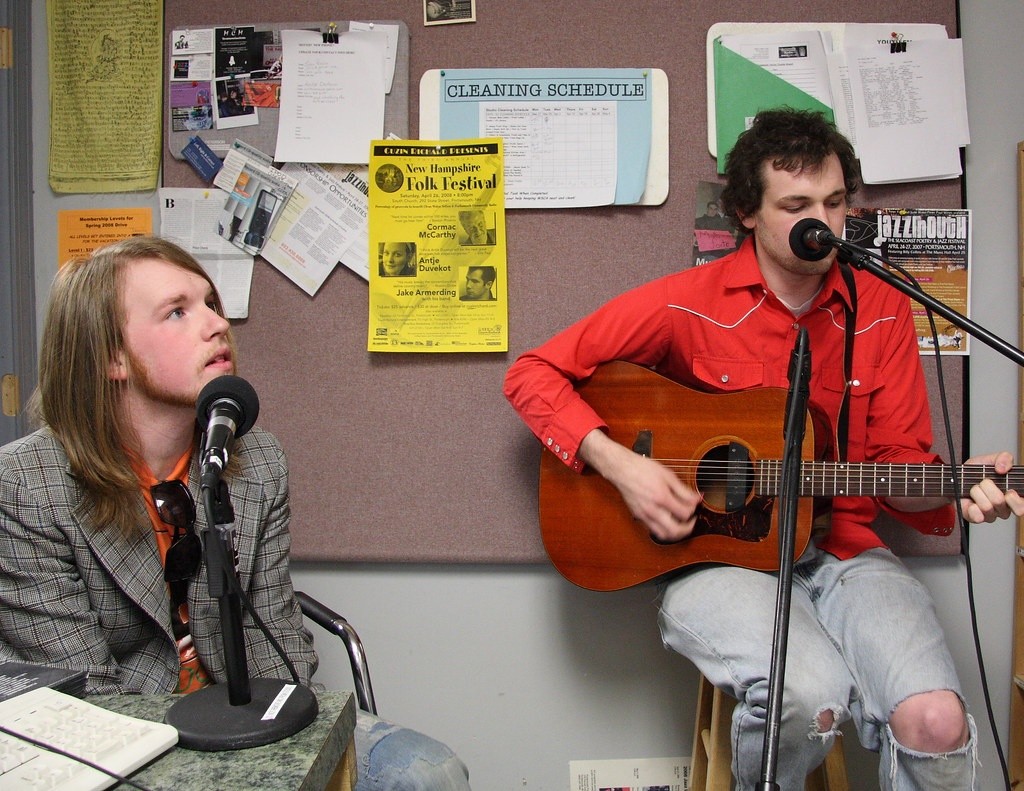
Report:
790,218,835,261
196,375,260,489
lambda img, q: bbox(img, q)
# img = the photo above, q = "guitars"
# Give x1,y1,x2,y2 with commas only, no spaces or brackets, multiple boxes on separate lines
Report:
537,360,1024,593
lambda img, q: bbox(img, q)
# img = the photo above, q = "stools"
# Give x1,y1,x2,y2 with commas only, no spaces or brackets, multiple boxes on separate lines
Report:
690,673,850,791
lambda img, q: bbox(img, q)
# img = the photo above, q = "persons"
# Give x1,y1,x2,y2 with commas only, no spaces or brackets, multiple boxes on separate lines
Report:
220,87,254,118
694,196,735,233
378,211,496,302
174,35,188,49
501,108,1024,791
0,236,471,791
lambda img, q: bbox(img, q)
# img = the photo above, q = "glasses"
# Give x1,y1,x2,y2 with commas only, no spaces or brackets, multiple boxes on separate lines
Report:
150,479,202,582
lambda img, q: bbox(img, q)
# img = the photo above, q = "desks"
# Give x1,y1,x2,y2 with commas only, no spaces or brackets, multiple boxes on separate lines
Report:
87,691,359,791
